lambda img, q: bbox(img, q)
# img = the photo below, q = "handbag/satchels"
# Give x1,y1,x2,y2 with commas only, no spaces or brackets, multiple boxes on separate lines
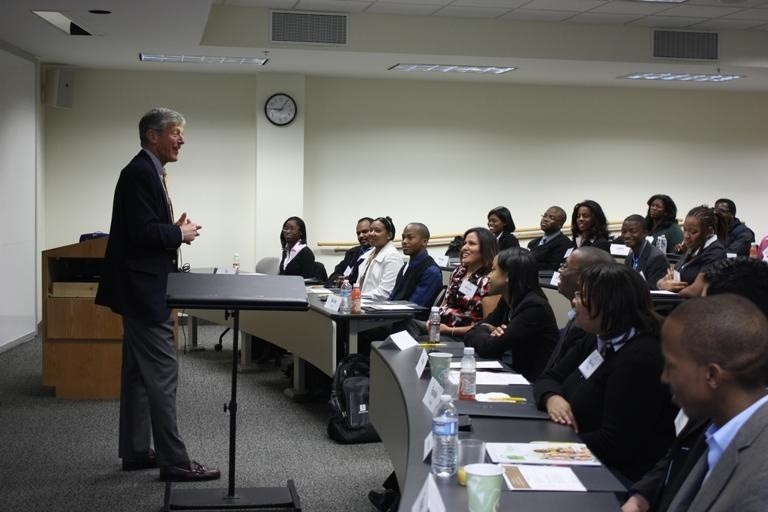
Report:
446,236,464,258
327,421,382,444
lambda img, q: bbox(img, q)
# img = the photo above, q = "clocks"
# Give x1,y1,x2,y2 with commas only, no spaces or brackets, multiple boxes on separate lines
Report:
264,92,298,126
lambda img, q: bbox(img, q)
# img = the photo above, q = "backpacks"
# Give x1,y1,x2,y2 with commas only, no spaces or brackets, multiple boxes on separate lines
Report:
330,352,370,429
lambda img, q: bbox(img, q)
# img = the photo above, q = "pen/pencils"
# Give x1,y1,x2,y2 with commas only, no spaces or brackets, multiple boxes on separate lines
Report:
489,397,528,401
416,344,448,347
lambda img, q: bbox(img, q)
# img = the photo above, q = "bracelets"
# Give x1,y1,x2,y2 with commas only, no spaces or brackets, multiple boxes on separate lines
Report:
451,326,456,336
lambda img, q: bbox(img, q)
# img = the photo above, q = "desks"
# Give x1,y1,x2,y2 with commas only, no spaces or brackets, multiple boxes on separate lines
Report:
160,273,310,512
432,251,576,329
366,333,625,511
180,288,415,429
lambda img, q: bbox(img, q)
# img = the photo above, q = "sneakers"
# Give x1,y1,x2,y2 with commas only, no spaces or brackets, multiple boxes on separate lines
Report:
368,490,396,511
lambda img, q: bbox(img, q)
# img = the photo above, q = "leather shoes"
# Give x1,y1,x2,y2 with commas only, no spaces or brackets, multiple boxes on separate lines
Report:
160,460,220,481
123,450,157,469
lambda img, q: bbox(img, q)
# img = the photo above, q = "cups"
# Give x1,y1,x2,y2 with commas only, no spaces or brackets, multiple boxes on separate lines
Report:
464,463,505,512
429,353,453,389
457,439,486,486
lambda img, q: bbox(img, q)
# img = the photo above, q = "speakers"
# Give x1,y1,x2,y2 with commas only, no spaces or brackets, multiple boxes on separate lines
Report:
43,66,77,112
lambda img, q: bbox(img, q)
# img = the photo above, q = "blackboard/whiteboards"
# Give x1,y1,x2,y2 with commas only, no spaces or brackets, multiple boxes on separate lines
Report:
0,38,42,361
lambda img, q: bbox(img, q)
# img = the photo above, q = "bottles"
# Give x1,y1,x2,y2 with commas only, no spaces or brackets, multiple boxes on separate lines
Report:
233,253,240,274
749,243,758,257
352,283,361,313
432,395,459,478
429,307,440,343
459,347,477,401
340,280,352,314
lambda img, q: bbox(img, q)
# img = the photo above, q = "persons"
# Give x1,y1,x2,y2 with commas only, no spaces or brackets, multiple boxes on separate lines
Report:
369,246,561,510
541,246,616,375
360,223,443,353
94,106,220,480
488,195,764,306
328,218,375,289
240,216,316,361
351,218,404,299
621,294,767,512
533,265,667,477
413,228,499,338
